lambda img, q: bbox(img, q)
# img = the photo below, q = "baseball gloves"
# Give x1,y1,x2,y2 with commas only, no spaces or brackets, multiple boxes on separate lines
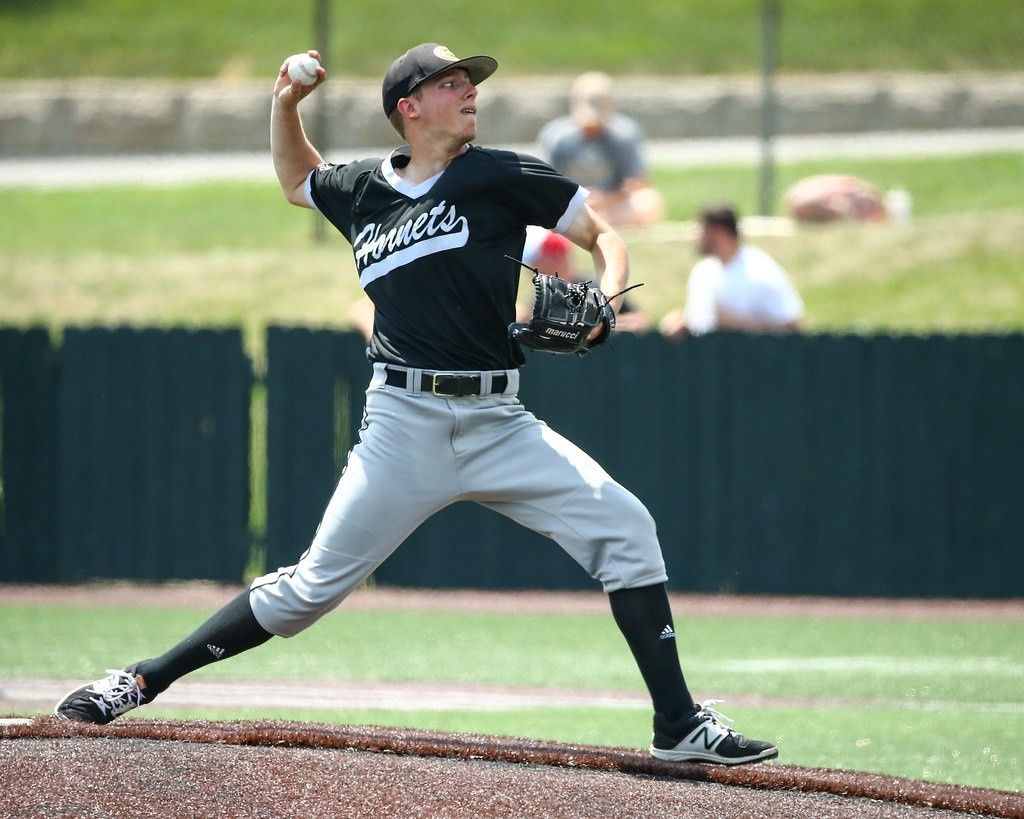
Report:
508,273,616,355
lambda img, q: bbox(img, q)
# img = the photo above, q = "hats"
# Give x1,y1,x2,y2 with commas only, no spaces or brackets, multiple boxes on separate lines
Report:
382,42,498,119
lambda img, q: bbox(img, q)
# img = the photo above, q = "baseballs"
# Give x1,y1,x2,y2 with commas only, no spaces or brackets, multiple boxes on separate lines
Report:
288,53,320,86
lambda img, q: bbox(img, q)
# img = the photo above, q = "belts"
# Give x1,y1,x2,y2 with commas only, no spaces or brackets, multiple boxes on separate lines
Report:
384,367,507,397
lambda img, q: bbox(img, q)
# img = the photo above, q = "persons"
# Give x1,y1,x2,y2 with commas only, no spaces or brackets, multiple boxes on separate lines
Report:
655,201,805,333
52,42,779,767
515,234,651,338
527,68,664,226
784,171,911,224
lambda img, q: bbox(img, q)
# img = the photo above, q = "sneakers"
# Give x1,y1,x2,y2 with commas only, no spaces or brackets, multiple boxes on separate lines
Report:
55,660,155,724
648,699,778,764
